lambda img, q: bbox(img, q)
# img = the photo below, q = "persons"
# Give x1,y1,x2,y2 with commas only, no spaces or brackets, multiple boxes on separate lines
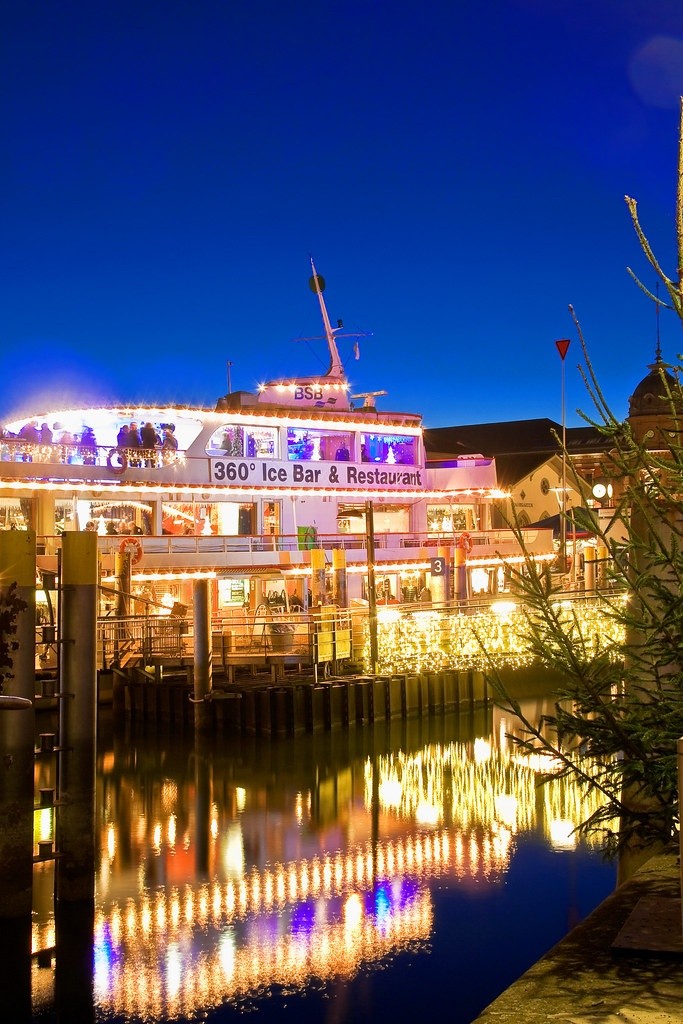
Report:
293,435,369,463
0,501,217,535
17,418,179,468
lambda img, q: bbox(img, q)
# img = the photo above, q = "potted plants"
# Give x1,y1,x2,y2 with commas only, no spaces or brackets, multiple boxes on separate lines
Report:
268,621,296,652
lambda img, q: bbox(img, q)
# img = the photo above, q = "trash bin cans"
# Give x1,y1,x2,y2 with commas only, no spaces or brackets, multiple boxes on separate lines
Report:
267,623,296,652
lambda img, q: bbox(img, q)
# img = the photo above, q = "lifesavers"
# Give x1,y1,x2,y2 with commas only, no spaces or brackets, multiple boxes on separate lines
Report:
120,538,143,565
458,532,473,553
106,449,129,475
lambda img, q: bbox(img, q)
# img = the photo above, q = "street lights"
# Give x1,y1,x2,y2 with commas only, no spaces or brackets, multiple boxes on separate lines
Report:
544,484,574,550
334,499,379,674
